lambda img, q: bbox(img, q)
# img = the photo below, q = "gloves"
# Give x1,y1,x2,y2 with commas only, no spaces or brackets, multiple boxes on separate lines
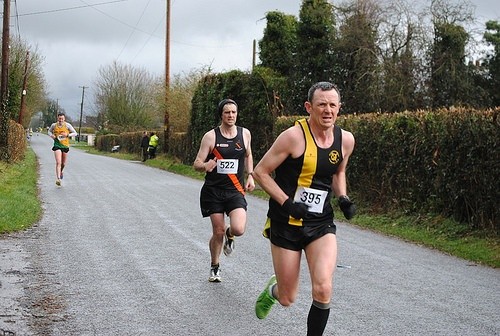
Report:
282,197,312,221
338,195,358,220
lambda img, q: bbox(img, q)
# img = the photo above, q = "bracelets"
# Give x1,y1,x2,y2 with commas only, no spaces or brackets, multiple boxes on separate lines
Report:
203,163,207,171
337,195,350,205
247,173,253,179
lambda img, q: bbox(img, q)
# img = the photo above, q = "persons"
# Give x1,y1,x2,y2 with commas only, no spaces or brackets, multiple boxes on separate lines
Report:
193,99,256,283
48,113,77,186
141,130,159,162
251,82,360,336
29,126,33,141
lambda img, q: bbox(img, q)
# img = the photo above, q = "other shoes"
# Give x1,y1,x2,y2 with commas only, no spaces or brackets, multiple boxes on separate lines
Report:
60,170,63,179
56,179,62,186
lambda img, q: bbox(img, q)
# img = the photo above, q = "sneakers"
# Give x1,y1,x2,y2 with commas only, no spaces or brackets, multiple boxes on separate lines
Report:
255,275,278,320
223,225,235,257
207,262,222,282
